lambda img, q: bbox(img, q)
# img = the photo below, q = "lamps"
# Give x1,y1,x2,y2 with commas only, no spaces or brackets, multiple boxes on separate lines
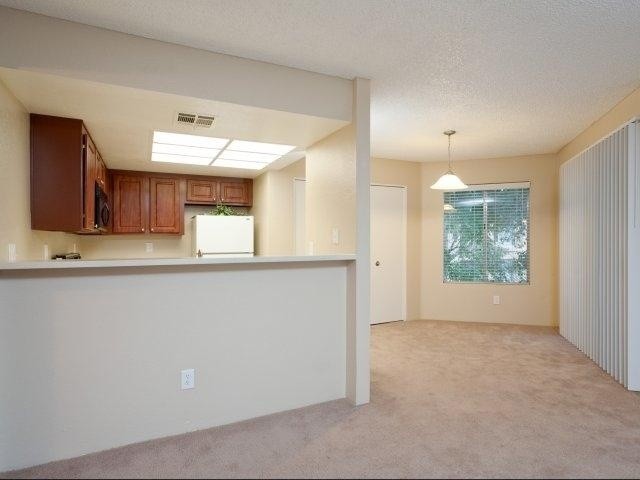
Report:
430,130,469,190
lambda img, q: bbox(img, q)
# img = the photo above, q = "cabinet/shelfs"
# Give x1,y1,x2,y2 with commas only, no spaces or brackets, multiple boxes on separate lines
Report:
30,113,253,235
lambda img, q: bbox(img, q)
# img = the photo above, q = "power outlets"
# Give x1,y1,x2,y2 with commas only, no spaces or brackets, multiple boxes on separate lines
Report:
182,369,195,390
